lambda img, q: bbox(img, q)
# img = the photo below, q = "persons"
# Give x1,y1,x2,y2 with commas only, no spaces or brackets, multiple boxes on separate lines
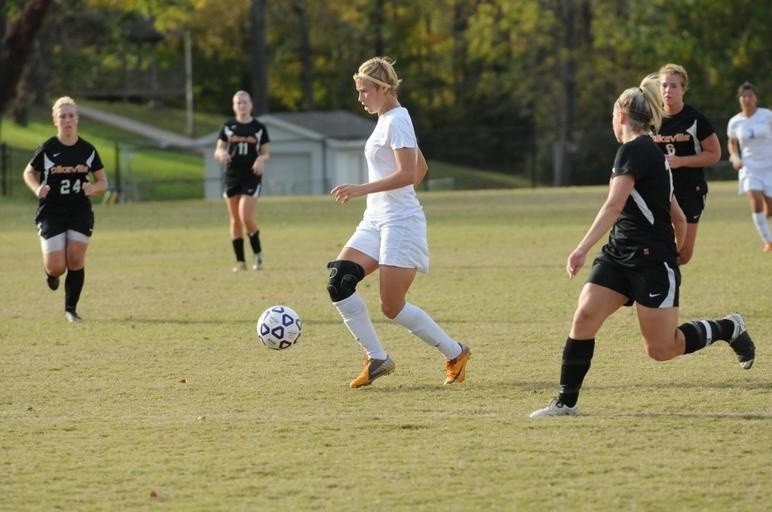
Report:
650,64,723,268
326,58,474,390
726,81,772,253
22,96,110,325
527,74,757,419
213,90,271,274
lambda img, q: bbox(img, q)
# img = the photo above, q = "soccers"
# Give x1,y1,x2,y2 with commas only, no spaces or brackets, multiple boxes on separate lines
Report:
257,306,303,349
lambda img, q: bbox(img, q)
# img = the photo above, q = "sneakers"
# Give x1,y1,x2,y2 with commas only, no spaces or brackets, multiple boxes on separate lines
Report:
348,357,395,391
729,316,756,369
251,253,264,272
44,271,61,292
526,400,582,418
441,342,471,386
232,261,247,273
64,309,81,324
